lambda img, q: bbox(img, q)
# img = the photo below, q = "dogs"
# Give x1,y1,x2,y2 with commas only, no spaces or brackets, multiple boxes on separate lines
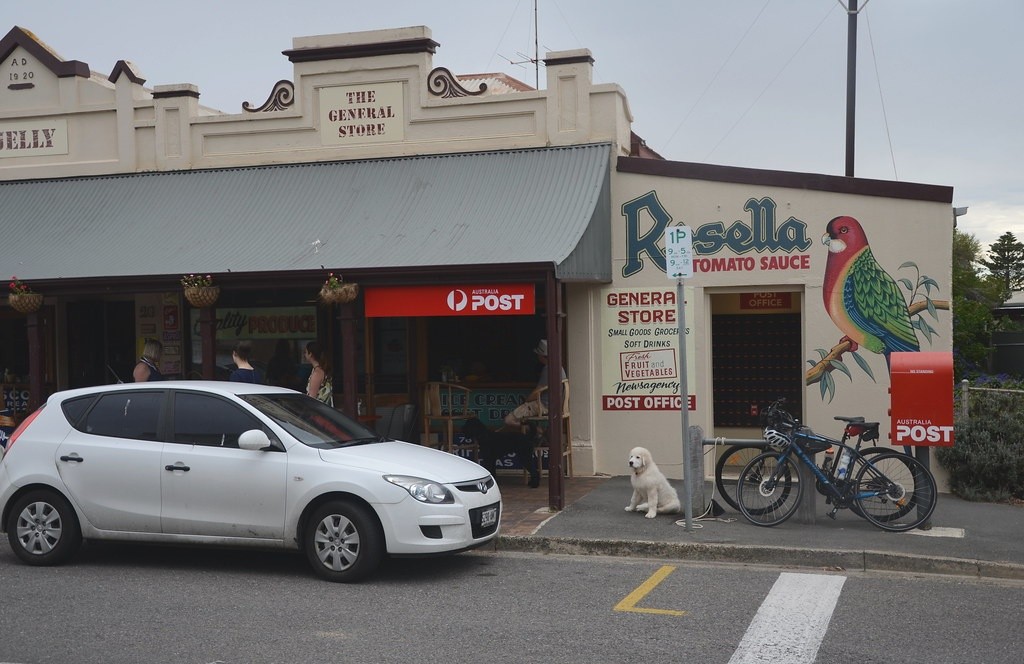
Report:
625,446,680,519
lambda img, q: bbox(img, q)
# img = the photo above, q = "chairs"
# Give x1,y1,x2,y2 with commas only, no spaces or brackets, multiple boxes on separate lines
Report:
417,380,479,455
519,379,574,485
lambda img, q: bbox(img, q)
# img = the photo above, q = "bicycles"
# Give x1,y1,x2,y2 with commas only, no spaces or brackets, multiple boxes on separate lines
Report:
736,410,938,531
713,397,919,521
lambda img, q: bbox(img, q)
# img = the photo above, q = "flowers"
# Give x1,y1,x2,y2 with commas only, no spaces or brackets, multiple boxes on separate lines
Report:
8,276,35,295
180,274,216,287
322,272,344,292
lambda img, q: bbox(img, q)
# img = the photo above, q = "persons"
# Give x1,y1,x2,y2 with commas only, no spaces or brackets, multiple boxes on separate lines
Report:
227,340,265,385
503,340,567,438
304,342,336,409
130,339,165,383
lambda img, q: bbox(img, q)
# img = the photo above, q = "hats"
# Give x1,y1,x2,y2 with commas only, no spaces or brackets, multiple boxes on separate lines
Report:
532,339,548,356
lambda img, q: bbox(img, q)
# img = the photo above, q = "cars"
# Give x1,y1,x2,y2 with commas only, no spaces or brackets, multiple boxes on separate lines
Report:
1,365,505,584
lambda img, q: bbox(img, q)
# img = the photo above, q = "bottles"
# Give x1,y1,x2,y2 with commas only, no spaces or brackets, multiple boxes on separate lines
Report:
823,447,834,473
833,452,851,480
